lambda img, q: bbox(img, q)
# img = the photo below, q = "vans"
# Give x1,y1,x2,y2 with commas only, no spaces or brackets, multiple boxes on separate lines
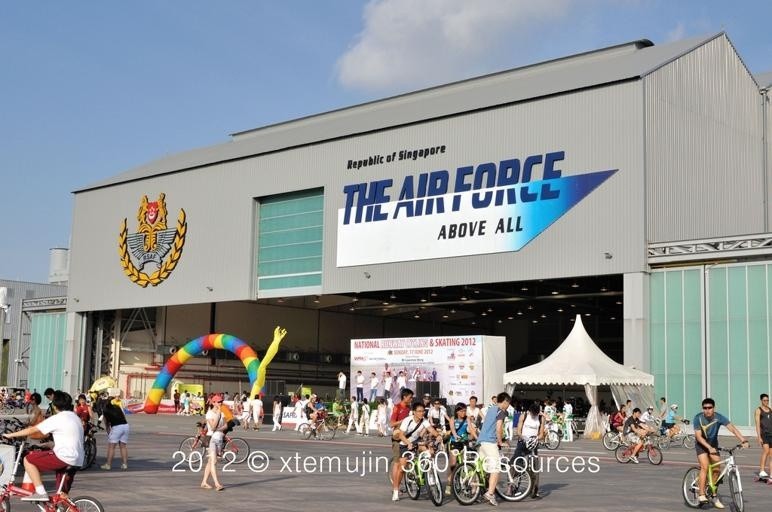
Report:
0,386,25,409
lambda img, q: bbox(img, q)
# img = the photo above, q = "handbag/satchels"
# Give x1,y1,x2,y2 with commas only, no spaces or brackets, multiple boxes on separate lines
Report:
443,432,452,444
201,436,211,448
232,418,241,427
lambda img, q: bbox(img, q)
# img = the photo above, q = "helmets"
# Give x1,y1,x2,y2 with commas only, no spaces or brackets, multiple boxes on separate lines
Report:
526,436,539,452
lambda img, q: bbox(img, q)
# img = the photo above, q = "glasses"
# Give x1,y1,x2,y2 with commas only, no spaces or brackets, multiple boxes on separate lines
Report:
415,398,467,413
703,406,714,409
28,395,51,402
761,399,769,402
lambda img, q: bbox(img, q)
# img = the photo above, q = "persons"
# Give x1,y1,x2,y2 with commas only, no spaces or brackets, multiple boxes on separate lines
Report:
396,371,407,392
173,389,265,491
694,397,750,509
355,370,366,402
290,389,685,507
380,363,437,382
271,395,282,431
383,372,392,402
369,372,379,403
337,370,347,402
755,393,772,479
0,388,129,511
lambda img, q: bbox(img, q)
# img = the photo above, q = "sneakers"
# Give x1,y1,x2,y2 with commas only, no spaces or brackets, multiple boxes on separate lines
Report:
2,424,771,503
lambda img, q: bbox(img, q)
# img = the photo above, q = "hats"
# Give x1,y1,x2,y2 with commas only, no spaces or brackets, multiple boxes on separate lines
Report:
671,404,678,409
206,395,223,404
647,406,654,411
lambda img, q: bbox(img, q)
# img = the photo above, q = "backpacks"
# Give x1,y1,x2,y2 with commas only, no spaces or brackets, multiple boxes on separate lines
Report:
609,411,622,425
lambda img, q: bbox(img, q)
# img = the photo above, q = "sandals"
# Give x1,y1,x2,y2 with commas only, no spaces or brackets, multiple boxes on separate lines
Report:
199,482,224,492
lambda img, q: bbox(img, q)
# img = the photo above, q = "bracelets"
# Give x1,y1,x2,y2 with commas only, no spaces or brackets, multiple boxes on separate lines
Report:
742,440,749,444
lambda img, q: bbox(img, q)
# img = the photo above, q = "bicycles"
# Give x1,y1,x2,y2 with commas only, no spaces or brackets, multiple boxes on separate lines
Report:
0,417,105,470
682,440,749,512
602,418,696,466
388,413,580,506
0,392,17,415
297,414,337,441
0,436,105,512
179,422,250,464
325,404,358,432
